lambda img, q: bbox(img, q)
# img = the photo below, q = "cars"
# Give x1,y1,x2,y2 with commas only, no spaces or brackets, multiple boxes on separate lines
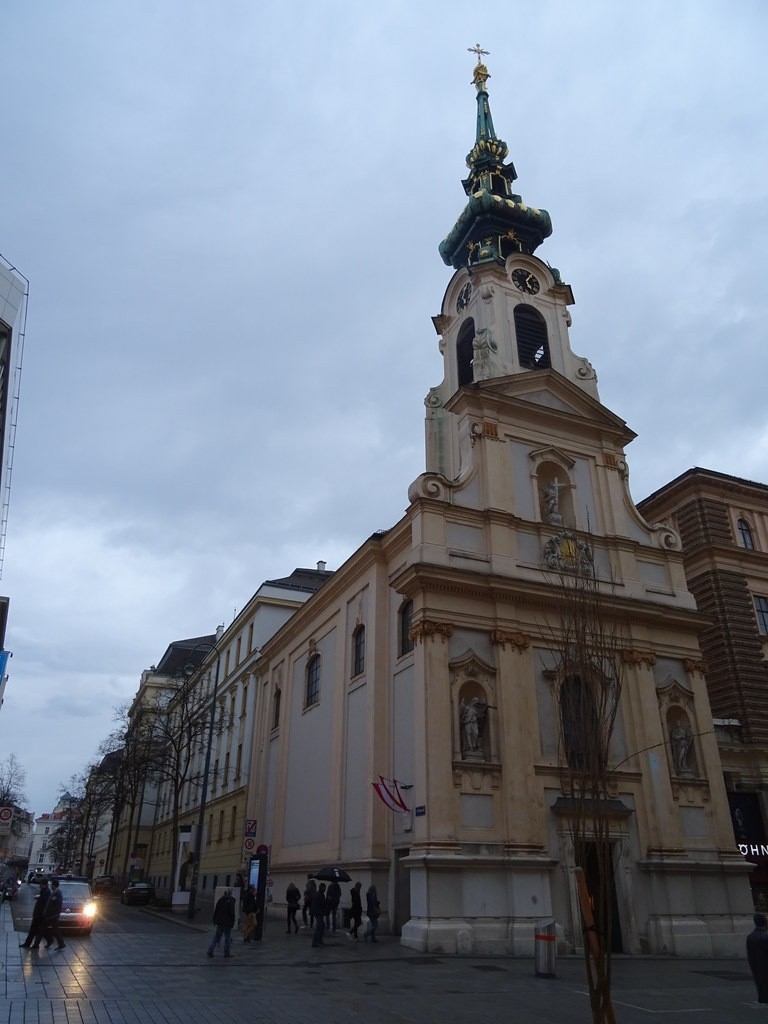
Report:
91,874,117,893
121,880,155,906
33,870,99,935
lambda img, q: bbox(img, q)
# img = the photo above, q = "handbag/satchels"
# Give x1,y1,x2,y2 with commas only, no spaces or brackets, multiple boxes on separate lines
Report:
374,905,381,917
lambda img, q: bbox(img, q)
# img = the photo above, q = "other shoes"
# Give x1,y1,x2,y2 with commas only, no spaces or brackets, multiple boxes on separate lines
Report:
346,932,353,941
301,926,307,928
207,952,214,957
286,931,291,933
354,936,363,943
55,943,65,950
19,943,30,948
45,940,53,948
224,954,233,958
371,940,378,943
312,944,321,948
31,945,39,949
295,926,298,934
244,936,250,942
364,933,368,942
320,943,324,945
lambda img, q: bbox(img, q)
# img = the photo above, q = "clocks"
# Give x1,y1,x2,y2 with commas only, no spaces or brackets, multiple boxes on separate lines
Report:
456,283,473,314
511,269,540,295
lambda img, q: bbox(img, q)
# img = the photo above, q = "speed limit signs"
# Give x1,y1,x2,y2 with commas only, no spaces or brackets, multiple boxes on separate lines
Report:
0,805,15,836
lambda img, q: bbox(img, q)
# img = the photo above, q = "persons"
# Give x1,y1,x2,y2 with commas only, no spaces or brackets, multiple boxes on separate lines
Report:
19,872,66,951
669,720,693,768
460,696,489,751
210,889,236,959
543,480,558,514
242,873,383,947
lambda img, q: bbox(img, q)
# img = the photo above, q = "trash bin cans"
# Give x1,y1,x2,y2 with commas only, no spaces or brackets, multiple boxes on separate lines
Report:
534,918,558,979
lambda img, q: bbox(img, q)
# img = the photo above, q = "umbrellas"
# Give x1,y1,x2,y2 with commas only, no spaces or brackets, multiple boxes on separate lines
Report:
313,867,353,882
